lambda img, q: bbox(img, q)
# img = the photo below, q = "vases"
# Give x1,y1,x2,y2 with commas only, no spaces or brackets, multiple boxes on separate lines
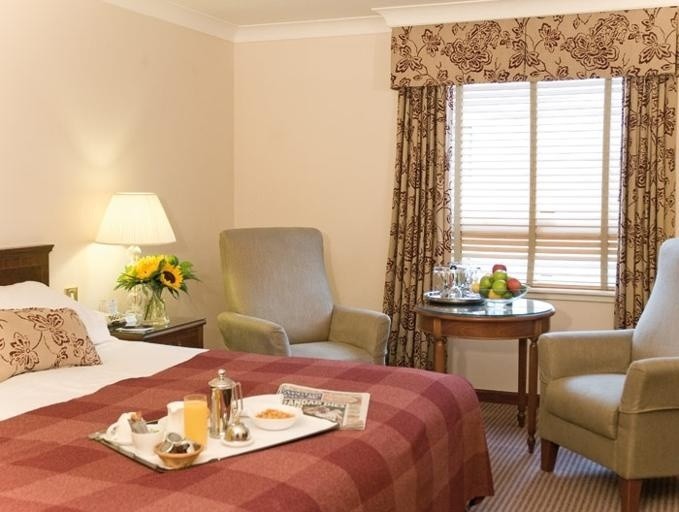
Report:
138,285,171,325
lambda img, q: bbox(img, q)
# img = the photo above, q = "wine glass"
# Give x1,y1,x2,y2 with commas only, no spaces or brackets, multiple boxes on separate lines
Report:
433,263,483,297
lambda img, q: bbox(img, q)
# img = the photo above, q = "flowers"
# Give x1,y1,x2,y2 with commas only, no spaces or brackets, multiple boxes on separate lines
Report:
112,253,202,299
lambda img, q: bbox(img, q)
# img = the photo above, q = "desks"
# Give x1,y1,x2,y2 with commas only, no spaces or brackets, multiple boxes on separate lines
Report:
414,298,556,454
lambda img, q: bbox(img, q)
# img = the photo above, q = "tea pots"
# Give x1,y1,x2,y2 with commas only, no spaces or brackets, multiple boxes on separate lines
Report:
207,368,244,442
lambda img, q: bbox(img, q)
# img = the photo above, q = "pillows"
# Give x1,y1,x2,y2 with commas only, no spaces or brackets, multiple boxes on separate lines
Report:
0,278,111,347
0,309,103,382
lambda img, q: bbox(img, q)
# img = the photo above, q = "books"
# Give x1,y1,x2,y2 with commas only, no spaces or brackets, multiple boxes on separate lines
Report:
276,382,374,432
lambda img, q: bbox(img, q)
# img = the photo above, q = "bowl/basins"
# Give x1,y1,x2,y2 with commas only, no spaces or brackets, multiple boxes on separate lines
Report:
152,440,204,465
473,285,529,305
248,402,304,432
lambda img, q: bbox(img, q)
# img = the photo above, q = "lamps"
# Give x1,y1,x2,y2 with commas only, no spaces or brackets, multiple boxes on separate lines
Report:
94,191,175,319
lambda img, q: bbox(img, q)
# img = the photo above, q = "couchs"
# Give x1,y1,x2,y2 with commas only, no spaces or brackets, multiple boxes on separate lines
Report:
216,227,390,364
538,238,677,511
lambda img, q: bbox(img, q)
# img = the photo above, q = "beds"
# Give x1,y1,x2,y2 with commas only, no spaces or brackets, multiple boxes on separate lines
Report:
0,243,495,512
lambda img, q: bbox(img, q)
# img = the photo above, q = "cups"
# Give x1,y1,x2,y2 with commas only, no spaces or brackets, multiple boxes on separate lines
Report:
133,423,169,452
184,393,209,448
165,400,186,428
107,411,145,448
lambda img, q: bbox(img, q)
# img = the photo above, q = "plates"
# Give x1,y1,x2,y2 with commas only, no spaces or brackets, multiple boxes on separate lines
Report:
222,435,254,447
422,290,484,305
157,415,170,429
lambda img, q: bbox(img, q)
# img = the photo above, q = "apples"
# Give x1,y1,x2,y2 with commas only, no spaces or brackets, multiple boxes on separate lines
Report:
471,264,522,299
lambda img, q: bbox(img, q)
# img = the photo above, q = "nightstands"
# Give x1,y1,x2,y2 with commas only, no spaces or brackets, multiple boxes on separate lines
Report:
106,314,206,351
